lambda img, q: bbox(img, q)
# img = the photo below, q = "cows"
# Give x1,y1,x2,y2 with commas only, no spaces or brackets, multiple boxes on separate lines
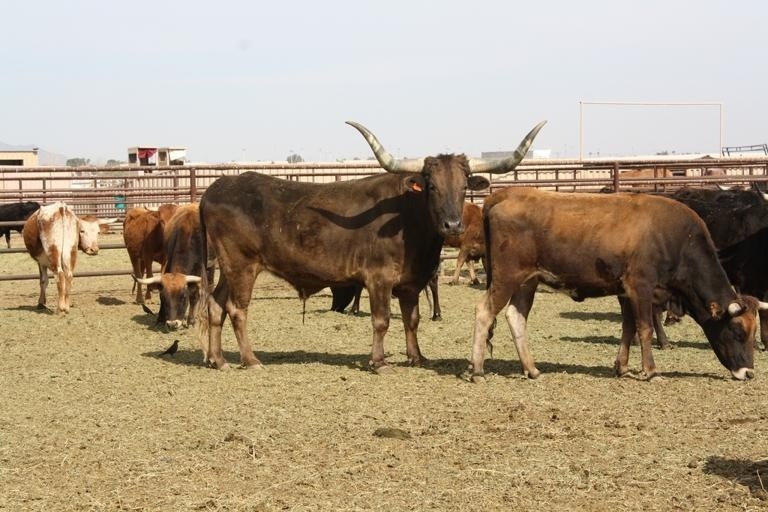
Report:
191,118,549,376
122,203,165,304
329,180,767,387
21,199,118,317
0,199,41,249
130,202,217,334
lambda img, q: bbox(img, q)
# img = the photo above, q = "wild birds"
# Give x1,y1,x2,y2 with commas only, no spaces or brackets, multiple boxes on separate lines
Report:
158,339,180,359
140,304,156,320
